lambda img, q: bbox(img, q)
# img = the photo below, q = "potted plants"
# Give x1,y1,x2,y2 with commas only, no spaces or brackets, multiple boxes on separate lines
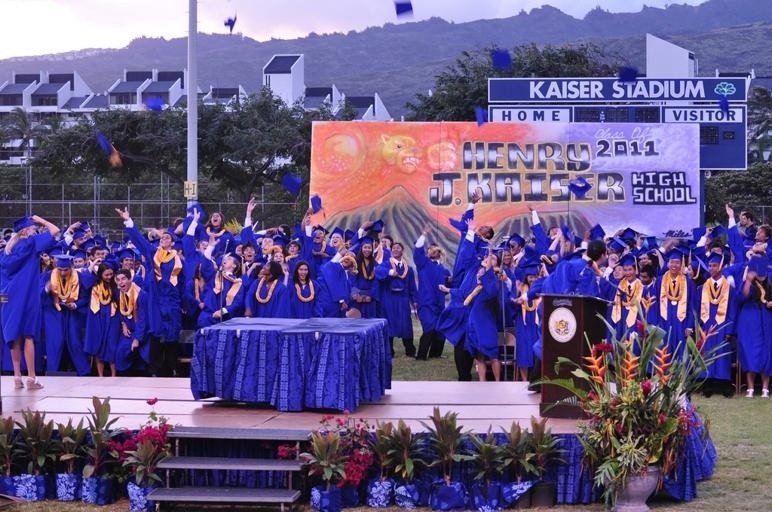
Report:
299,433,343,512
0,396,119,504
366,406,570,512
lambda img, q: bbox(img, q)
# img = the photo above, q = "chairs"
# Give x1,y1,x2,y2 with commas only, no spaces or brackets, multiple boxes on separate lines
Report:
484,324,517,381
739,361,762,395
177,330,193,364
729,336,739,395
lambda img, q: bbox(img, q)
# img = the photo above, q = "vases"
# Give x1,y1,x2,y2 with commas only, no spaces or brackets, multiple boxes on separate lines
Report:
338,485,357,507
127,482,158,512
611,465,660,512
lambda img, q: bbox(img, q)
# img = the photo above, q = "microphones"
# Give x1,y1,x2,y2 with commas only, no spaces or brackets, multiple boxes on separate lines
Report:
225,239,229,253
497,250,505,279
576,259,594,283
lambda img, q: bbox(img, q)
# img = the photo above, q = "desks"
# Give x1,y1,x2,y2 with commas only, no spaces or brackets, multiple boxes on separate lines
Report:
191,318,391,413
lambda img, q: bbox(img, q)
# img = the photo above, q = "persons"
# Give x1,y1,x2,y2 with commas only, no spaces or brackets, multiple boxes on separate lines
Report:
412,222,450,360
437,190,772,397
0,198,417,390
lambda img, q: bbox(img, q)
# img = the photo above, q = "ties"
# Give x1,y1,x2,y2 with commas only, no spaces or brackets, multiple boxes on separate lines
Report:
714,282,717,289
673,281,676,287
125,294,129,304
63,278,66,287
629,285,631,293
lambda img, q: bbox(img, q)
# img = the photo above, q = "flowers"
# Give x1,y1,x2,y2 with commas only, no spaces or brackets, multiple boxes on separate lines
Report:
525,319,734,511
106,398,174,487
278,409,374,490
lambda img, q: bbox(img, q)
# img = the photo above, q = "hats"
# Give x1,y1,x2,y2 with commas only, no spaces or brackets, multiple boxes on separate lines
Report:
619,67,637,82
506,224,768,281
0,217,384,268
719,94,731,111
475,108,488,124
283,174,321,214
224,18,236,31
145,96,162,110
570,177,590,195
492,48,511,69
396,3,412,15
97,135,121,167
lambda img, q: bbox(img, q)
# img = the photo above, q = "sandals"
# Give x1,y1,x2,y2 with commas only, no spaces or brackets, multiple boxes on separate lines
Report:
27,377,43,389
14,377,23,389
